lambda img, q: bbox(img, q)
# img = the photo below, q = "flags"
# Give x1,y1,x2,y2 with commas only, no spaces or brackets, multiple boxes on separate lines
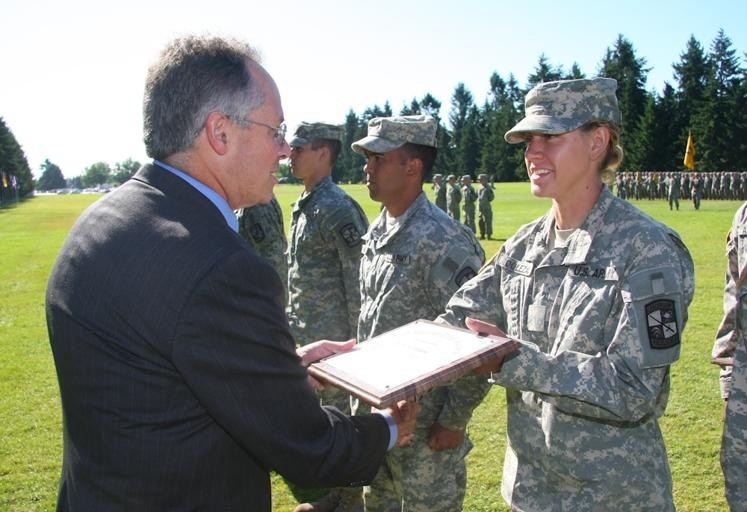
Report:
682,133,694,170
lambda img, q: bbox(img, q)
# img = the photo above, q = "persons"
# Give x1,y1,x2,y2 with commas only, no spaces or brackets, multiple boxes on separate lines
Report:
461,174,477,236
711,197,745,508
284,123,365,512
44,33,413,512
233,192,288,320
488,172,496,190
349,115,489,511
446,173,459,221
393,79,693,511
431,171,446,214
477,174,492,239
598,161,746,210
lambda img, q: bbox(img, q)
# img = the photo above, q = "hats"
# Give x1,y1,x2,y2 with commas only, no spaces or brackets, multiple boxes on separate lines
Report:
290,123,341,147
504,77,622,144
351,114,438,154
432,174,487,181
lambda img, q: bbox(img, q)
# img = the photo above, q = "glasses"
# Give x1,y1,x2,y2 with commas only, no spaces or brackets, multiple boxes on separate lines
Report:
195,115,286,146
